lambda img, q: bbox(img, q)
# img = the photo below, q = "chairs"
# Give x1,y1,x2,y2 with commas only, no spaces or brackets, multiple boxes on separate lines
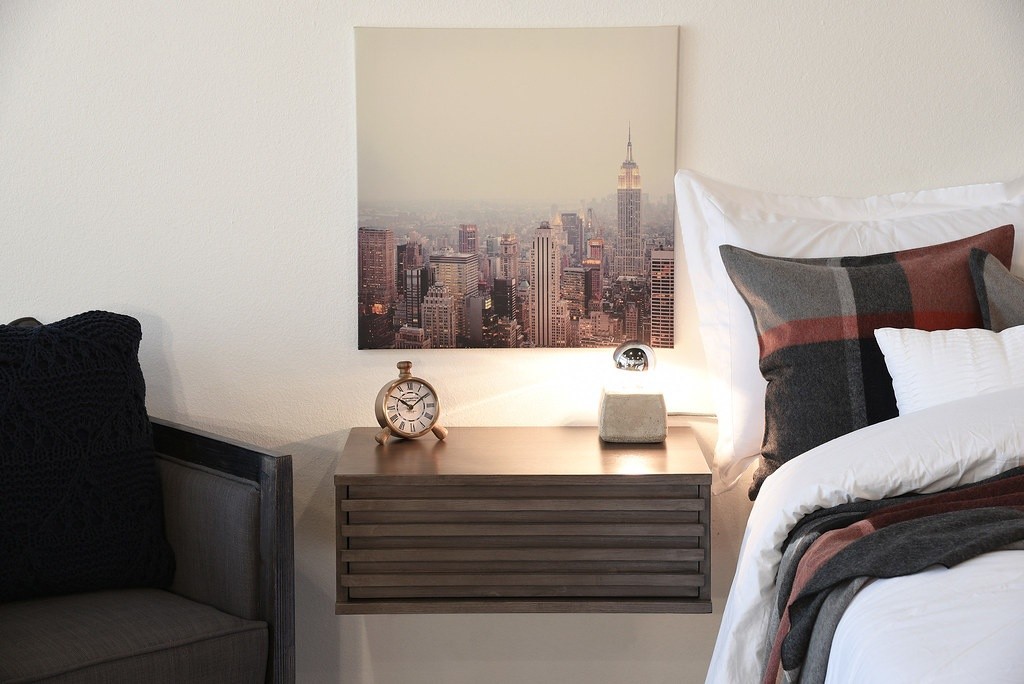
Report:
0,316,298,684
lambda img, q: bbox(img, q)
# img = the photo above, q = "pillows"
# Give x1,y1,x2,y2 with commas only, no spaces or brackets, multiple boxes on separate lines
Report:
0,310,176,603
672,168,1023,503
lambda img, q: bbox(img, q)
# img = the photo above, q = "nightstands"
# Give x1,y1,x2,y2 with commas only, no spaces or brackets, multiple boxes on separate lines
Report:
332,425,713,615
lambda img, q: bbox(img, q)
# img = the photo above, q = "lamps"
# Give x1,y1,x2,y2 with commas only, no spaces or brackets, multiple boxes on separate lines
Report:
374,361,450,447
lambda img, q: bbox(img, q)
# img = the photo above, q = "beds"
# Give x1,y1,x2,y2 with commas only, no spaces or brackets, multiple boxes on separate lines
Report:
701,383,1024,683
